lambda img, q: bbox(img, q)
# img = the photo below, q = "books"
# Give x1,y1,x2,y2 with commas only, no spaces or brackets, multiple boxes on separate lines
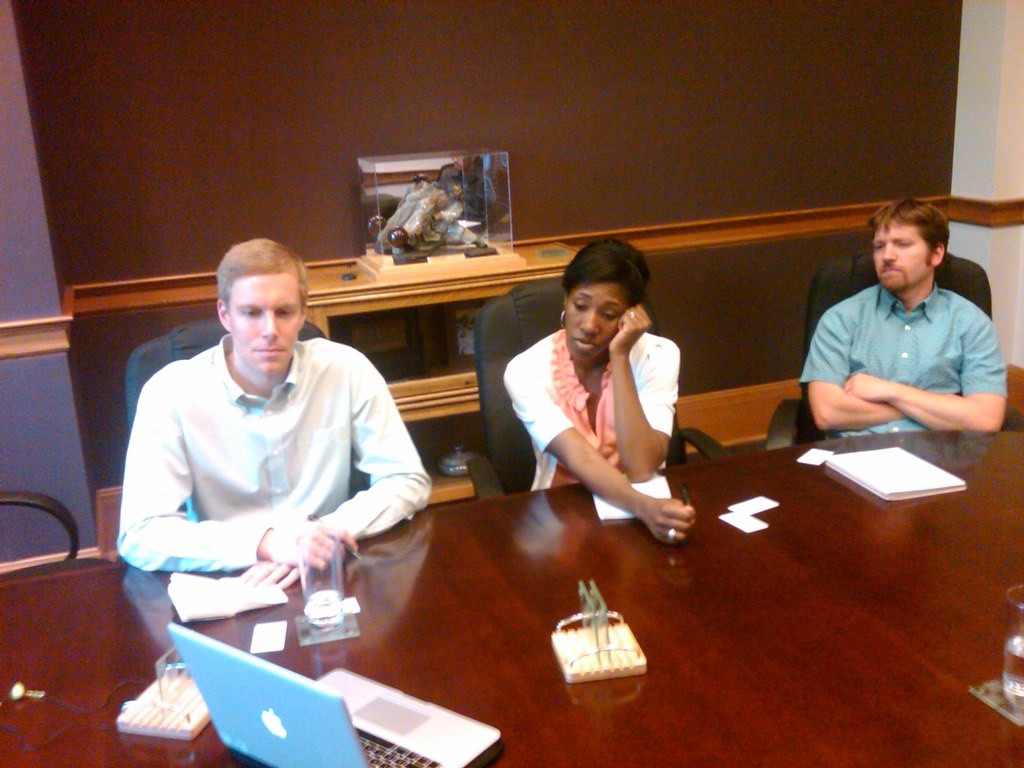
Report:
168,572,289,623
117,673,211,740
825,447,967,500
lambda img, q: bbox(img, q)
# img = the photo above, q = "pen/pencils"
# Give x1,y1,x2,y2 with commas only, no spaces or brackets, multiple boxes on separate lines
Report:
306,514,363,564
681,483,693,506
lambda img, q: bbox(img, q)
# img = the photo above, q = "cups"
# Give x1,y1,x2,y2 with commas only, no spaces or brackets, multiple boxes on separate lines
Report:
1003,585,1024,709
296,530,344,630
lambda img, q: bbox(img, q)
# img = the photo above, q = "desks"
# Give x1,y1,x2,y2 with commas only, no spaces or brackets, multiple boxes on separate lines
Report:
0,429,1024,768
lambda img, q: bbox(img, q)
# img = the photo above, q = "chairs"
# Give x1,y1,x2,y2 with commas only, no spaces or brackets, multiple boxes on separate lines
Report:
0,489,112,581
467,275,730,499
124,315,370,499
766,244,1024,449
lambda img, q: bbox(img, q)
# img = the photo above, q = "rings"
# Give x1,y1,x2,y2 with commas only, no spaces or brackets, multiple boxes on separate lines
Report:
665,528,676,541
629,311,635,319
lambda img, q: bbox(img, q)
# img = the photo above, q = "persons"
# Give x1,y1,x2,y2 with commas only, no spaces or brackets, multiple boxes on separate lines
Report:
503,238,694,543
118,239,430,591
440,136,496,222
798,197,1006,437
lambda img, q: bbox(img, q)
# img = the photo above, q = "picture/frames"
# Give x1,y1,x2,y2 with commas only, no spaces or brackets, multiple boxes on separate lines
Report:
447,298,488,364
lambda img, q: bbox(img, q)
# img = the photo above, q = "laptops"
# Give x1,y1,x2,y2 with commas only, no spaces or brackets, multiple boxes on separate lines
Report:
165,621,503,768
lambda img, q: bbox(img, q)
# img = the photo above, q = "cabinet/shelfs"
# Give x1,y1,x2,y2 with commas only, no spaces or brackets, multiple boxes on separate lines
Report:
304,241,579,504
